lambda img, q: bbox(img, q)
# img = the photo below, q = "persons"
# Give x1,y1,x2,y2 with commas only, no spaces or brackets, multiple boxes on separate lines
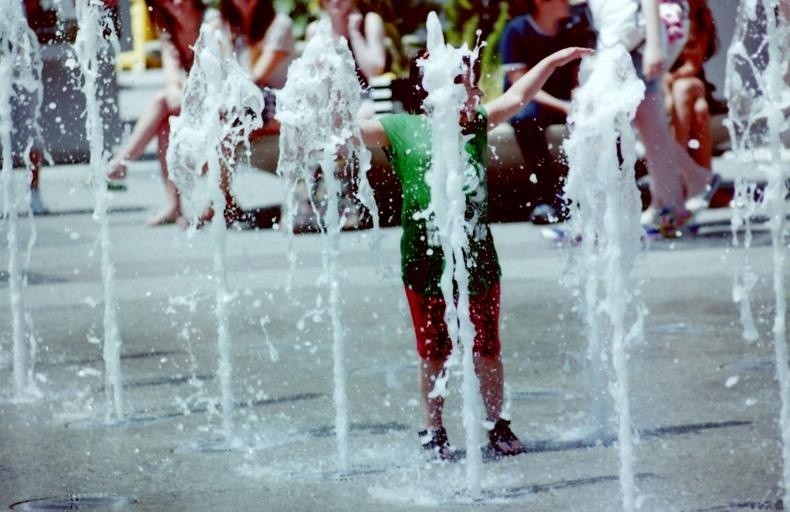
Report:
340,36,601,459
632,87,724,211
94,0,297,234
274,0,397,239
639,0,721,171
534,1,693,255
134,0,252,229
496,1,604,225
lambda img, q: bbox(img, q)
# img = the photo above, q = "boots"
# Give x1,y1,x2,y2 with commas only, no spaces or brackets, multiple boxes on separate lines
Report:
485,417,528,456
418,427,456,461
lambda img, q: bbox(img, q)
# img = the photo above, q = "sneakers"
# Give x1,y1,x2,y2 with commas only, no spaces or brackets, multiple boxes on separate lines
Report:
87,174,129,191
146,198,359,235
642,174,722,235
531,205,557,224
